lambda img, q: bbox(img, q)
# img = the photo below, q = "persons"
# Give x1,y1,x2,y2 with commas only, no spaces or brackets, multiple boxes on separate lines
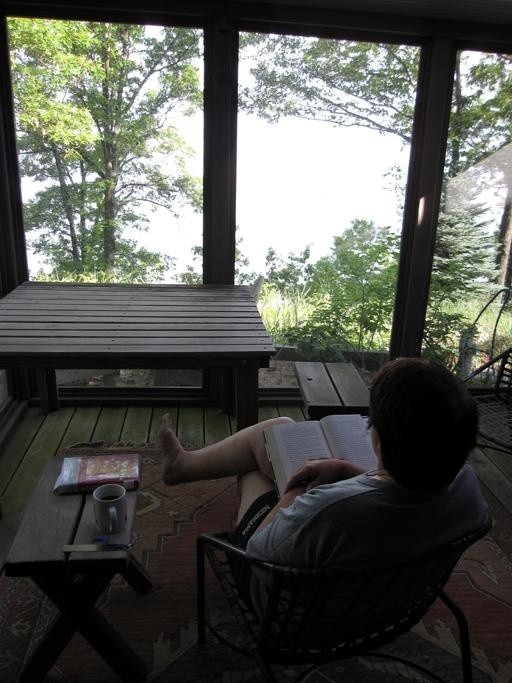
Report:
157,354,491,641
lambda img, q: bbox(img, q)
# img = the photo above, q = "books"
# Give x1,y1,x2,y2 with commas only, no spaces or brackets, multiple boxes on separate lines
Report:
263,412,378,500
53,452,142,496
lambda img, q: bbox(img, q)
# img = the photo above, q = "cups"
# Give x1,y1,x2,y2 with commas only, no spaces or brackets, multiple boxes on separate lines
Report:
93,484,126,534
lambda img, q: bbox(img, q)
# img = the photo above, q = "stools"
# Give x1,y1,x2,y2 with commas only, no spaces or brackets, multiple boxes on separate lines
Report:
294,361,374,413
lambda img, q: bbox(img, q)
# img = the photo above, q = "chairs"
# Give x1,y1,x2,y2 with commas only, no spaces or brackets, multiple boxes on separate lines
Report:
197,512,492,683
459,347,512,454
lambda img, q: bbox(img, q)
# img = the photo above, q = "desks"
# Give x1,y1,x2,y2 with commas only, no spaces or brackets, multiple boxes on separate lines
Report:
3,456,152,683
0,281,277,435
